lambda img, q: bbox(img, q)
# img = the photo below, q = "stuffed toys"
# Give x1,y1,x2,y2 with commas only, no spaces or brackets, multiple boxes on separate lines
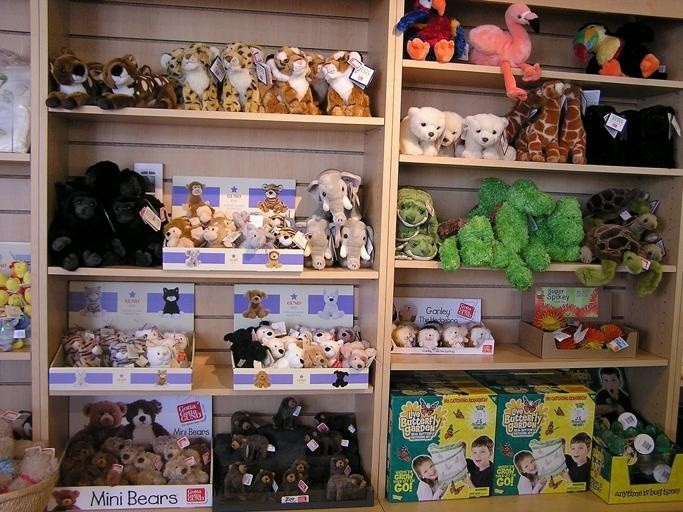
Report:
60,326,193,368
1,396,367,502
396,0,680,349
224,321,376,370
1,261,31,349
47,161,376,272
1,1,376,508
1,48,31,153
394,0,680,350
46,41,374,117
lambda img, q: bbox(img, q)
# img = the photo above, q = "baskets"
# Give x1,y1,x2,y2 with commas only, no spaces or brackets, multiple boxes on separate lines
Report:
0,440,57,512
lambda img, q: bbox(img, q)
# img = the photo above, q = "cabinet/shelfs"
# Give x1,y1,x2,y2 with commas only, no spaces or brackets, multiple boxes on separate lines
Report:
0,0,42,491
30,0,400,510
357,0,681,511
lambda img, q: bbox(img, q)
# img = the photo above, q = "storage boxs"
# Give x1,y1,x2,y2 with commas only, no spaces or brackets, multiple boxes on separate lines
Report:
384,368,498,504
465,367,598,497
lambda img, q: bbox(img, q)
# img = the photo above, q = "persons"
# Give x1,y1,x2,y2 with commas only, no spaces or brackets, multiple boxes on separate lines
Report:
413,435,494,501
594,368,630,405
514,433,592,494
